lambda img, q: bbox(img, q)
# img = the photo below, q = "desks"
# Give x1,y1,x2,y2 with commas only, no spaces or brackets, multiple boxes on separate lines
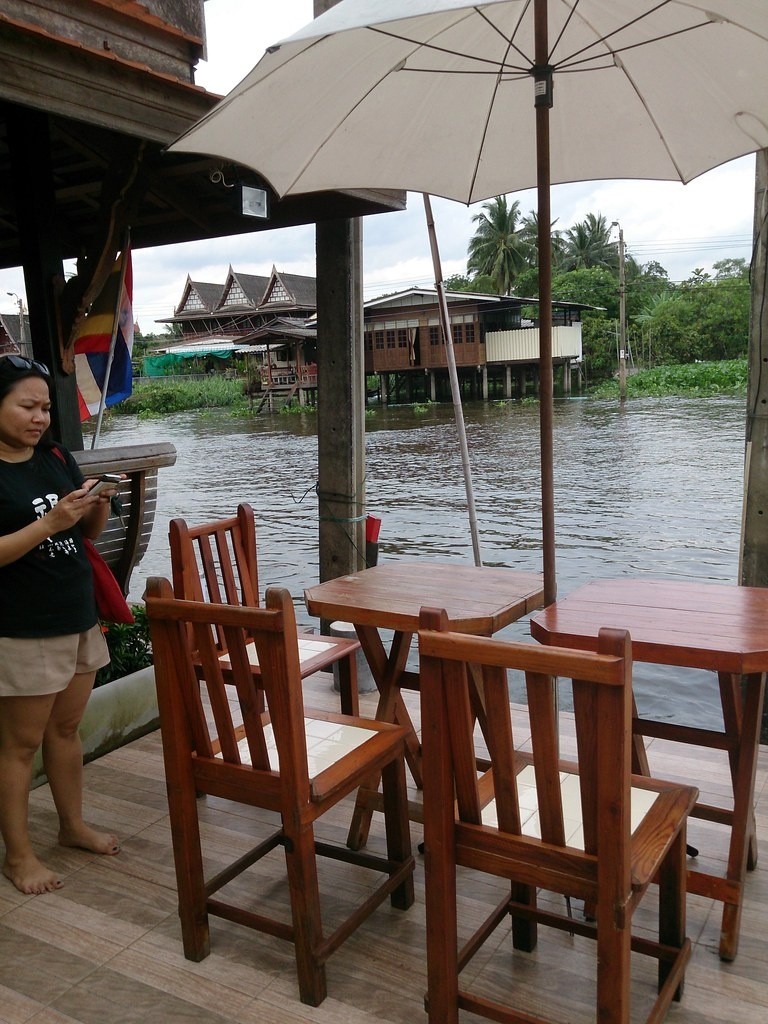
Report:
304,557,545,852
530,580,768,962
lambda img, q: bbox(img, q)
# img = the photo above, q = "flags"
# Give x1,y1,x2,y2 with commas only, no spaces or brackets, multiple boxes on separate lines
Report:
74,240,132,423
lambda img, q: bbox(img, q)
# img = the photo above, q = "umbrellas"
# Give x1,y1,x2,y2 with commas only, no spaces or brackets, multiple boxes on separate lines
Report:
160,0,768,602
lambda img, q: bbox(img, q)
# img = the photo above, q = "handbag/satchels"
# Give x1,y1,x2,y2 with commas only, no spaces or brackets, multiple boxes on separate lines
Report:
51,446,135,624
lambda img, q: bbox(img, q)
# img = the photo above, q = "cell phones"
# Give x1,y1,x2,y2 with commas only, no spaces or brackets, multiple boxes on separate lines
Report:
79,474,121,499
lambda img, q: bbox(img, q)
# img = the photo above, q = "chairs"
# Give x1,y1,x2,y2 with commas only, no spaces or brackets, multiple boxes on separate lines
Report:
144,577,416,1007
418,605,698,1024
166,504,362,798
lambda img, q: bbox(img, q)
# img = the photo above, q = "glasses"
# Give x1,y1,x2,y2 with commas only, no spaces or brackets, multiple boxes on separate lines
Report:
0,355,50,377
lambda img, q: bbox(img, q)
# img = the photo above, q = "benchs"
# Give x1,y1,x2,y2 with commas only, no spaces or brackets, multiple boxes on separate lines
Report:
68,441,176,595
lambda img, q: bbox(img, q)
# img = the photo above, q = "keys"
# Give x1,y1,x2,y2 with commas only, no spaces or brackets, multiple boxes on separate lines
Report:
111,496,126,532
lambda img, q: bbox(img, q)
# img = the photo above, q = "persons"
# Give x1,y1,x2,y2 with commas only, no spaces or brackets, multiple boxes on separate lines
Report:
0,355,127,894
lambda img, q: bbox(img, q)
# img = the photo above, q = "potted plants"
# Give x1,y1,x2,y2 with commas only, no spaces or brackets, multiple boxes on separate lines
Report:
28,603,161,815
